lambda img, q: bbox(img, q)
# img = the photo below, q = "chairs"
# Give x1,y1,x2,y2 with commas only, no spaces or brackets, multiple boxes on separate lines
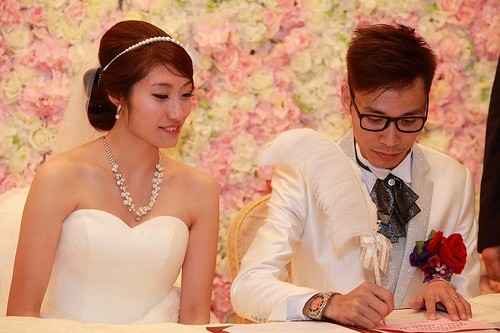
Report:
228,192,292,325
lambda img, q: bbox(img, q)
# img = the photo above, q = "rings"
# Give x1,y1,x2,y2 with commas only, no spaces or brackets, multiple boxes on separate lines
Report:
452,296,458,300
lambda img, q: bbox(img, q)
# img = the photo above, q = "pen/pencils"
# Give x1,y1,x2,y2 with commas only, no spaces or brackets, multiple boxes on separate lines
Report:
372,254,387,326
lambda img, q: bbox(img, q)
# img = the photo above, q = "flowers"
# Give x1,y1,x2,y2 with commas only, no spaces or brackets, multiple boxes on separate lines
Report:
408,228,468,282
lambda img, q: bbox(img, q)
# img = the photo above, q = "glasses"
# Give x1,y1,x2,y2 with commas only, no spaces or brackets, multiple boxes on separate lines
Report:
348,84,429,133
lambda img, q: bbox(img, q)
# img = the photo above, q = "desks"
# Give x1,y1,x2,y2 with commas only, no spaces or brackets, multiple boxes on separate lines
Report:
0,291,500,333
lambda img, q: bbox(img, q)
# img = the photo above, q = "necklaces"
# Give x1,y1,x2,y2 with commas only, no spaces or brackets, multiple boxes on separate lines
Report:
103,135,164,222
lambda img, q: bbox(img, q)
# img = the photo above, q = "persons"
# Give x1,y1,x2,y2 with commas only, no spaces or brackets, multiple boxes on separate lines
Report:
230,21,480,331
0,21,221,325
476,51,500,282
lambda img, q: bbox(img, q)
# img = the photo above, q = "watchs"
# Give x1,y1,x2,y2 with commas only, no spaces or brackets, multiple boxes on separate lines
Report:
308,292,342,321
